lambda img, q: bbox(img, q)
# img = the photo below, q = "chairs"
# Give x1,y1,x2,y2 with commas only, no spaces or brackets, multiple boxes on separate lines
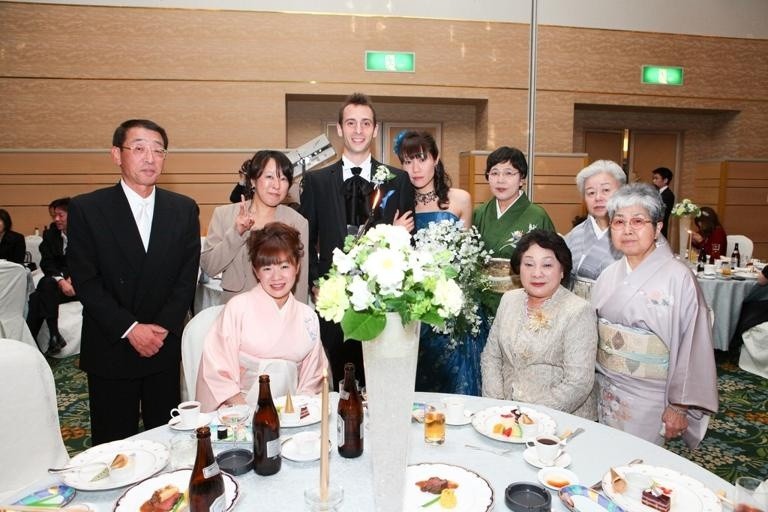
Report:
0,338,70,508
724,234,755,262
23,234,48,290
737,319,768,380
177,301,228,408
0,257,42,352
28,265,85,360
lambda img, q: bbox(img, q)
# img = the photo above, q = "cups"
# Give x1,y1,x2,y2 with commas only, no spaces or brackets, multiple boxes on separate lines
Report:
732,476,768,512
424,400,445,446
170,432,198,471
448,403,464,421
525,434,561,463
339,378,358,394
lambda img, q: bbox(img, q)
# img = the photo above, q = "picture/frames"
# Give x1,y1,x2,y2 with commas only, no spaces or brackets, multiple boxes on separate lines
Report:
382,118,443,176
317,115,384,173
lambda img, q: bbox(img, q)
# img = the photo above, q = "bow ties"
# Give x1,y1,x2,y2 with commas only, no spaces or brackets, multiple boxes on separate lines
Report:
341,166,376,229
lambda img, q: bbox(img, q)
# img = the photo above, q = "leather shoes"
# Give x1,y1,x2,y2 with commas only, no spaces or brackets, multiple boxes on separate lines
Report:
44,333,67,357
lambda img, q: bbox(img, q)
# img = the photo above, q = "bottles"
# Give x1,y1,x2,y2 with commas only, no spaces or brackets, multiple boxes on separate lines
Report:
42,225,48,234
302,483,346,511
189,427,227,512
34,226,40,236
336,362,364,459
252,375,282,476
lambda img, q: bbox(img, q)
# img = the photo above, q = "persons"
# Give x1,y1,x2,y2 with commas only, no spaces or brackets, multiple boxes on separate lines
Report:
300,93,416,394
651,167,768,373
194,220,335,413
480,228,598,423
588,181,719,452
0,198,80,356
392,129,494,397
62,120,202,447
564,159,670,298
470,145,556,333
199,149,311,311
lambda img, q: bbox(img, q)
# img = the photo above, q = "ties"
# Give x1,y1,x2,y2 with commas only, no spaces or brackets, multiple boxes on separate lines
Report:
138,201,150,254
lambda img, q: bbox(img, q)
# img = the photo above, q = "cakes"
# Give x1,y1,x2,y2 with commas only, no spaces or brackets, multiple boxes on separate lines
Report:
642,481,671,511
295,402,309,419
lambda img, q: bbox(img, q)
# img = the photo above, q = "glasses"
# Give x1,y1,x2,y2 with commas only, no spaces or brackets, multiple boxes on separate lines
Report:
609,216,654,231
120,144,169,161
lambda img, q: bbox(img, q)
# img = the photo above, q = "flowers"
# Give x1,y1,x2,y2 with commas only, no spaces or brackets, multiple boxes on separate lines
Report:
496,223,540,251
671,197,701,219
406,217,499,348
305,204,469,353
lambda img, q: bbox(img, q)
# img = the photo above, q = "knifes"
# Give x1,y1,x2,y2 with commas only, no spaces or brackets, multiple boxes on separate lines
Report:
589,459,644,489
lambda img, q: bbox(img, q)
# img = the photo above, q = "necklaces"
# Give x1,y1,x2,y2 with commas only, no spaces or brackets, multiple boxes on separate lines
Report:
527,307,536,315
415,190,438,205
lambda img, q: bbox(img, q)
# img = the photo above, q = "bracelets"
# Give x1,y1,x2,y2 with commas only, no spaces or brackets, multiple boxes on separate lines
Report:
668,404,689,415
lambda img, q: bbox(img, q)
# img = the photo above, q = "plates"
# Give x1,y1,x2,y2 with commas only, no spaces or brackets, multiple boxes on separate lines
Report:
471,405,557,443
444,408,474,426
557,483,625,512
523,448,574,469
674,242,768,280
10,485,76,508
56,438,170,491
168,391,332,444
601,465,723,512
282,429,334,462
537,467,579,491
404,460,497,512
112,468,242,512
410,401,436,422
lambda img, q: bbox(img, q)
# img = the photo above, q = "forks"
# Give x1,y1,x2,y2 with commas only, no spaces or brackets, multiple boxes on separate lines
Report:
558,427,586,450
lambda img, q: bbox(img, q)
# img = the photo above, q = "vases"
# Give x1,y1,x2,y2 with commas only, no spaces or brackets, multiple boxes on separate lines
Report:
677,212,692,265
357,308,423,512
